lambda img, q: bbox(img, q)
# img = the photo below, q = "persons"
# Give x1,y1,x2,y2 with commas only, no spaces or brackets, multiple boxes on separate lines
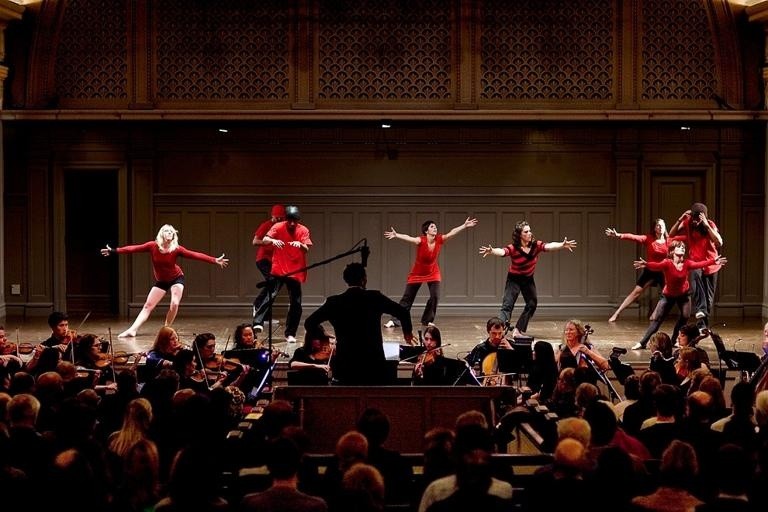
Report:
252,202,285,321
464,316,515,387
554,317,608,372
97,224,231,337
410,326,443,385
648,332,677,385
673,323,710,369
603,217,674,326
254,205,313,343
382,214,480,330
303,263,419,385
1,324,335,512
478,220,579,341
321,408,414,512
418,338,768,512
40,312,77,347
666,201,725,332
631,240,729,352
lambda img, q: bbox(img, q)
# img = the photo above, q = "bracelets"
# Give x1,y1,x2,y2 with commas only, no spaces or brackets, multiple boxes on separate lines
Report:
111,245,118,255
677,218,681,224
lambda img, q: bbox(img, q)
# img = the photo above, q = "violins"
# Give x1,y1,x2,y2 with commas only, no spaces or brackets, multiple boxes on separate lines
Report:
61,329,88,345
3,342,40,355
315,345,335,358
207,355,258,372
252,339,289,358
92,352,147,367
191,368,229,383
417,348,436,373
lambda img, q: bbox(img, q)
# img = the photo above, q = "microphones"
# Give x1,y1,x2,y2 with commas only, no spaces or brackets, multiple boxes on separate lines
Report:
418,329,422,347
361,240,370,268
734,338,742,352
708,322,726,333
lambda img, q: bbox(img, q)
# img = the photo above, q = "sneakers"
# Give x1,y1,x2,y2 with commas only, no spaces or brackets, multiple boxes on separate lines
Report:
286,336,296,342
253,325,263,334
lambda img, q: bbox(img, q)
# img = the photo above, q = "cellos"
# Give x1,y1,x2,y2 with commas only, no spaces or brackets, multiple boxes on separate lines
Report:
574,324,594,368
748,355,768,408
482,320,516,386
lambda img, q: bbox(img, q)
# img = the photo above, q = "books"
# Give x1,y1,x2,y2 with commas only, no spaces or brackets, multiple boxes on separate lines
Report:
381,340,402,362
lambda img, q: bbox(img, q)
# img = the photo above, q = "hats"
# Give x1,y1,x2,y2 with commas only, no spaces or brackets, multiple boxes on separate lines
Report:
271,204,302,221
